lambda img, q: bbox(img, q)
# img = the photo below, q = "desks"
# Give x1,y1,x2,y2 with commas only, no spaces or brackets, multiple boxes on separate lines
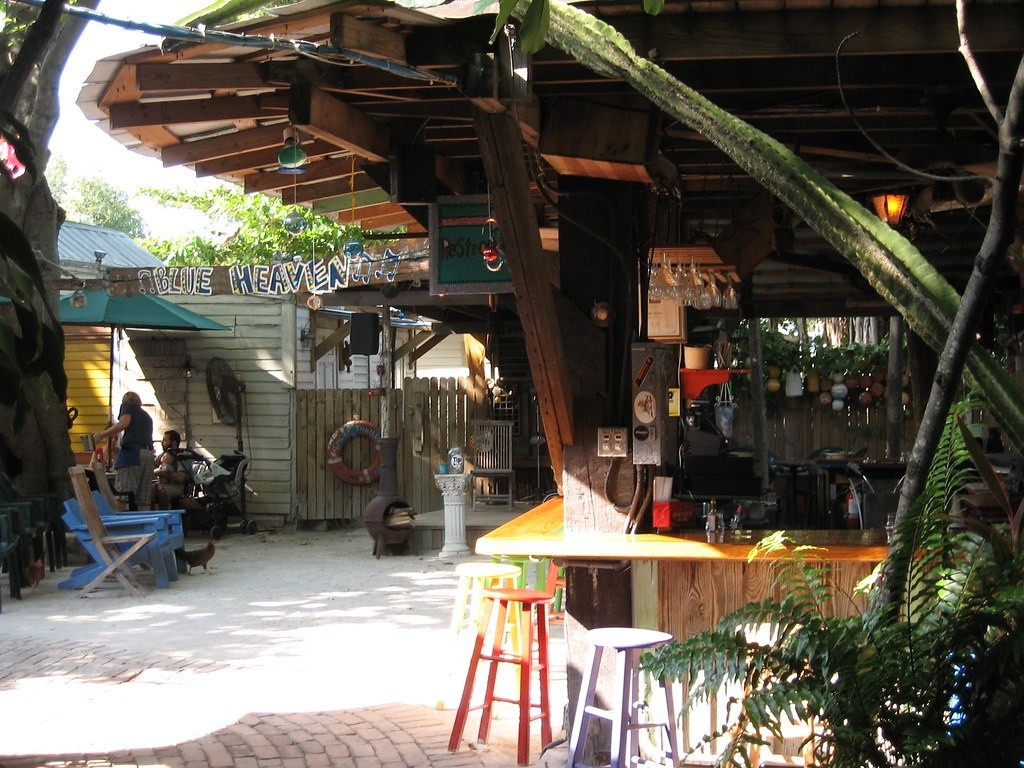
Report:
473,496,936,560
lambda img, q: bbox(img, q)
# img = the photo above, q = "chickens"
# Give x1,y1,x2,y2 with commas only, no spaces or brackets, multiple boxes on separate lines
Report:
174,538,221,576
25,556,44,593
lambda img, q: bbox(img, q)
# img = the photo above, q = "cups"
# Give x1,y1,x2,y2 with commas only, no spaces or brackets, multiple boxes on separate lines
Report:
80,434,96,452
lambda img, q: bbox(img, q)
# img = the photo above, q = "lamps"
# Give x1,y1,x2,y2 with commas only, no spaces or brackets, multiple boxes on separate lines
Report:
31,249,89,308
872,182,911,228
277,128,308,168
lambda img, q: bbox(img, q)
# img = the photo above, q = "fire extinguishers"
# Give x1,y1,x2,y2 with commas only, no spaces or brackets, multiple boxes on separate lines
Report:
842,482,863,530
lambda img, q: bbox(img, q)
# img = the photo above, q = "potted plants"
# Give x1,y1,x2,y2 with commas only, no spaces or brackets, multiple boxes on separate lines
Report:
734,320,911,411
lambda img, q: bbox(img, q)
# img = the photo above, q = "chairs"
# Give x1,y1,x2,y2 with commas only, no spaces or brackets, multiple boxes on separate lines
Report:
0,463,187,616
469,420,514,511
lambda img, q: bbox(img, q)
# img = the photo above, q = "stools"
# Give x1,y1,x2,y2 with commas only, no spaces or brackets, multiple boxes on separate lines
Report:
443,554,803,768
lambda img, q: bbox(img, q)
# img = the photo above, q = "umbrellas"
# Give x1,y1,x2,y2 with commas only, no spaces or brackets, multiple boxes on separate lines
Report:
59,289,233,470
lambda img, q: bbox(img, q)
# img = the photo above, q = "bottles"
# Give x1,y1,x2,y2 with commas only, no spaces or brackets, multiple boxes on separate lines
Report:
705,500,724,543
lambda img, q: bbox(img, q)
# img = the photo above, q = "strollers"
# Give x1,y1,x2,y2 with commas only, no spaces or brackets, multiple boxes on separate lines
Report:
168,445,259,541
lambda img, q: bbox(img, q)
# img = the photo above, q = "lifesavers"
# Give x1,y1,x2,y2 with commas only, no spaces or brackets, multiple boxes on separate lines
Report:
327,420,382,485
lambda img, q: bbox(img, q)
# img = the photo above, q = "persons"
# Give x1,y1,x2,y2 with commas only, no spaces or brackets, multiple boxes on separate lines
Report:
89,421,120,469
153,431,188,510
93,391,152,511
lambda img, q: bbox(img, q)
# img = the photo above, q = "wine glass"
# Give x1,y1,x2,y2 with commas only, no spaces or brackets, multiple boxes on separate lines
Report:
648,253,737,310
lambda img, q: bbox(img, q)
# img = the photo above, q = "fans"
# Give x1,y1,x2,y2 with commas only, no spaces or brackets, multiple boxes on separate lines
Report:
206,356,247,532
